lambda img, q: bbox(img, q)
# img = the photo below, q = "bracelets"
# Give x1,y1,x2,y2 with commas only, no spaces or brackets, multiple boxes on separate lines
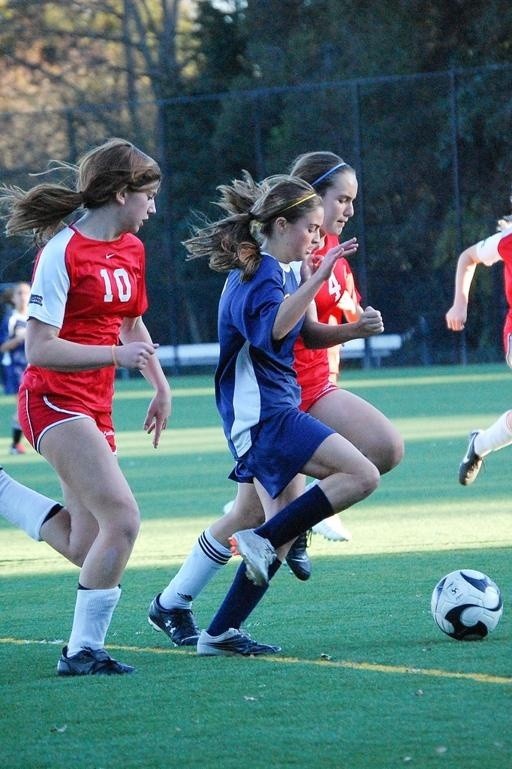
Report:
112,343,118,367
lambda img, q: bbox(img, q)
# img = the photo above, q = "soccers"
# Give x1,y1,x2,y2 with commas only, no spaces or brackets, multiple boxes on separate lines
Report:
430,569,503,641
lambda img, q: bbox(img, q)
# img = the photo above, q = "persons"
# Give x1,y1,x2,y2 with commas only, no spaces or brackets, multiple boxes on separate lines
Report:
2,281,30,454
182,166,382,653
147,148,407,648
444,222,512,488
2,140,175,675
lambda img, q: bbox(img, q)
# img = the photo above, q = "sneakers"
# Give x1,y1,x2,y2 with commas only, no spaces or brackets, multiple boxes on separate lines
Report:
145,590,202,648
287,526,317,581
10,443,27,455
303,479,352,544
458,429,485,487
194,627,284,658
54,644,134,676
227,527,277,590
